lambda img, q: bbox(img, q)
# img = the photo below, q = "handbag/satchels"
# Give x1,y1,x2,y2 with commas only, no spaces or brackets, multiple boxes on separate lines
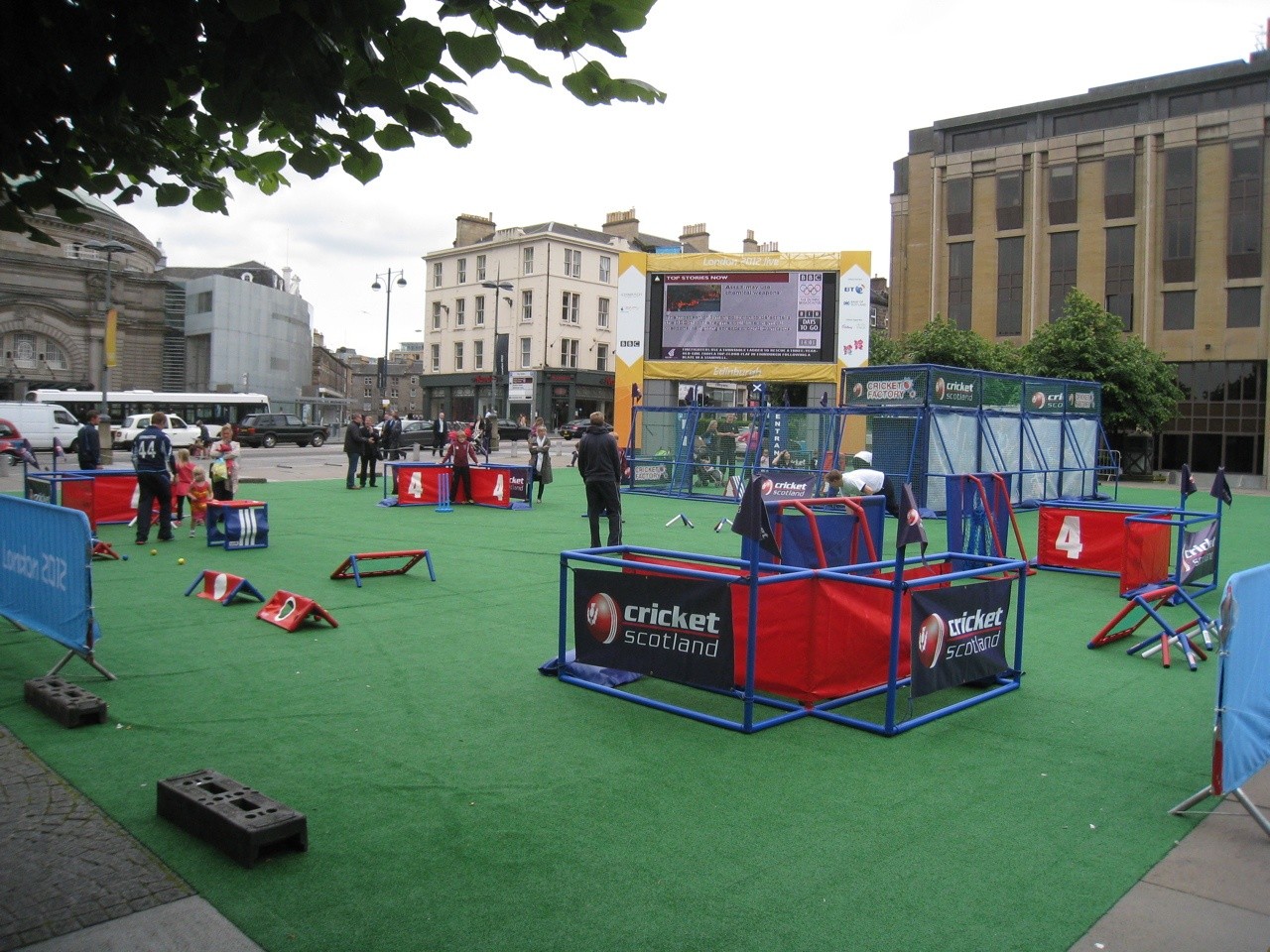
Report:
211,458,228,484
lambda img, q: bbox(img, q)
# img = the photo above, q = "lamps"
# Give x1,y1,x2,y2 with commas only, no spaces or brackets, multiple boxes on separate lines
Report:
503,297,512,307
439,305,449,314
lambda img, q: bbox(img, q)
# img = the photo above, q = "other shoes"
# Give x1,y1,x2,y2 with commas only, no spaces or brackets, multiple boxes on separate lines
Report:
360,484,365,487
376,450,383,461
433,452,435,456
158,535,174,541
195,518,204,526
135,539,143,544
347,485,360,489
468,499,474,503
445,500,451,503
190,531,195,537
382,456,387,459
370,485,378,487
566,464,573,467
441,456,445,457
404,453,406,459
572,451,576,454
537,499,541,503
388,458,399,461
175,521,182,526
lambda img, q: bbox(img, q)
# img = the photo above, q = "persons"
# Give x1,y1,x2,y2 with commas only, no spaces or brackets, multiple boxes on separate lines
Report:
470,408,492,447
566,442,579,467
717,413,740,480
431,412,448,457
521,424,553,504
174,419,241,537
852,451,873,470
824,468,899,519
516,413,544,436
737,423,758,480
771,449,797,469
78,409,102,470
131,411,179,544
608,431,627,523
577,411,622,548
439,431,482,505
698,420,718,469
343,411,407,490
760,449,769,472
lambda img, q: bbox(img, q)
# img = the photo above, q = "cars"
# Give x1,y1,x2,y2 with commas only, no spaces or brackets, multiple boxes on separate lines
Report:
373,418,434,449
558,419,613,440
112,412,210,449
0,417,25,466
233,413,331,449
497,418,531,441
735,425,769,457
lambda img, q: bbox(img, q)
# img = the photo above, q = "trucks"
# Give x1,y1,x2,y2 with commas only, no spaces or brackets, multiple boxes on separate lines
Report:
0,401,88,453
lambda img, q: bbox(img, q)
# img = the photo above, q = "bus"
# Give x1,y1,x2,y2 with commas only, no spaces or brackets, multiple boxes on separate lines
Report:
25,388,270,447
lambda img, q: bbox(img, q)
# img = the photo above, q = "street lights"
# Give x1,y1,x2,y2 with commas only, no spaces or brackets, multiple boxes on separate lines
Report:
371,267,408,422
481,259,514,451
83,239,136,465
